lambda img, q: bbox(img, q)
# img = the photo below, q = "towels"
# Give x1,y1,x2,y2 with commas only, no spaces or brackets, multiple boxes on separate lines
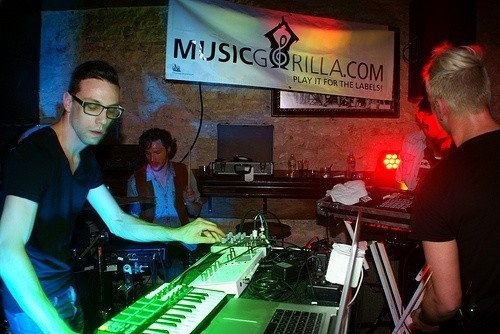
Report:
325,179,369,206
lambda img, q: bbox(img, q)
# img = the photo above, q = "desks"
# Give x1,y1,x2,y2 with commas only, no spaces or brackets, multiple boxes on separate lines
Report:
192,170,354,199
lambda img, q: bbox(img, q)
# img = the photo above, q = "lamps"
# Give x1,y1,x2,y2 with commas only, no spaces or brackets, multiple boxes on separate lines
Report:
375,150,401,171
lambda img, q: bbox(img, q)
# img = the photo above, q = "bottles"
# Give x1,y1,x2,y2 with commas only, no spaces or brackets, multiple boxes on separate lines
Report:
347,153,356,173
288,154,296,176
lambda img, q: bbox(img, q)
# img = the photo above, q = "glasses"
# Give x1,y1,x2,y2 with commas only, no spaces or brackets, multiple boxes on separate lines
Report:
72,95,124,119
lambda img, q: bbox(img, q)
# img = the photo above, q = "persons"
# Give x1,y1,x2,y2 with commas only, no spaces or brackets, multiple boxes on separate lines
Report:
126,126,202,284
410,42,500,334
396,96,456,194
0,60,228,334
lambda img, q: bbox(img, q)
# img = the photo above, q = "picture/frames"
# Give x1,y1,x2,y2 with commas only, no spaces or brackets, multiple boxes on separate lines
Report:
271,26,400,119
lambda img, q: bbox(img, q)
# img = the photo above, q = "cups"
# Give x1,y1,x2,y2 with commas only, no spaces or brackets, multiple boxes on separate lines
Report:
321,167,334,178
298,160,308,177
199,165,210,176
